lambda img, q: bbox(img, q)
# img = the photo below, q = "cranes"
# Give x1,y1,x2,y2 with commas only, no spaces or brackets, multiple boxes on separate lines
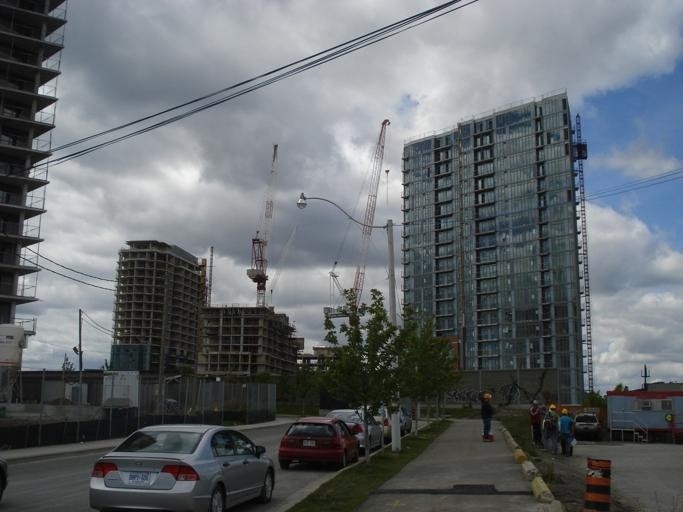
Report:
245,144,279,307
322,119,391,355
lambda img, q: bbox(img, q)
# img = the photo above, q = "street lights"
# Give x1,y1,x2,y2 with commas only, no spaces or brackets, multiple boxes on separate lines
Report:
162,375,183,424
297,194,402,453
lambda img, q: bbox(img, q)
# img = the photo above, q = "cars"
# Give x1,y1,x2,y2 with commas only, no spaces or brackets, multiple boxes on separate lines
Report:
574,414,601,442
0,455,10,500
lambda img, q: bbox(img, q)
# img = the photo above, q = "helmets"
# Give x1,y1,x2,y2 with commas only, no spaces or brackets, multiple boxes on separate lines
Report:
532,400,568,415
482,392,492,400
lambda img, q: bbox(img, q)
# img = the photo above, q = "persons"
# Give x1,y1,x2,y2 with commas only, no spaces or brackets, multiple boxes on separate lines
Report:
529,399,544,448
559,407,574,455
540,404,558,455
479,390,497,441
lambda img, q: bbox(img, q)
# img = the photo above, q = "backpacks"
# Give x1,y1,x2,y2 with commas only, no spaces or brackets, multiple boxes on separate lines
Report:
544,412,554,430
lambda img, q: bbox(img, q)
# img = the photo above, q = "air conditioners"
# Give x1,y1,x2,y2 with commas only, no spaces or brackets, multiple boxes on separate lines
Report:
641,400,651,408
